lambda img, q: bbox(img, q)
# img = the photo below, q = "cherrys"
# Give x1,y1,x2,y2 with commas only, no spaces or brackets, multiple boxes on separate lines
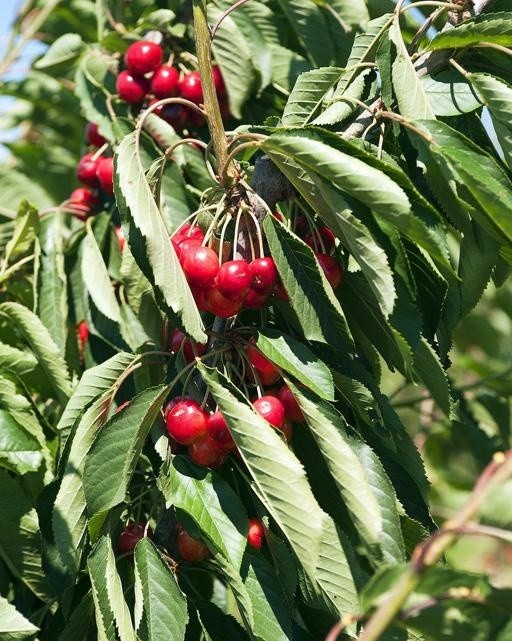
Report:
67,39,346,562
